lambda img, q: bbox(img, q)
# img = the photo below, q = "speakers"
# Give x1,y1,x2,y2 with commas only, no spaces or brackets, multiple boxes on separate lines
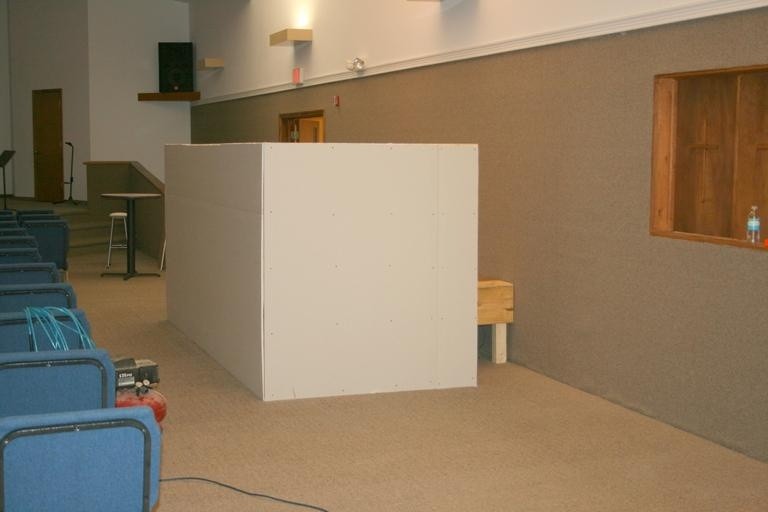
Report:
158,42,193,92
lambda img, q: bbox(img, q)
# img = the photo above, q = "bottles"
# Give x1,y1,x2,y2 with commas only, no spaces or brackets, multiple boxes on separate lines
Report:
745,205,761,243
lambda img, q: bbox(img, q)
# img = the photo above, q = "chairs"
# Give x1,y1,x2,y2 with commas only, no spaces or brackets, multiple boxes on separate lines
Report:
1,206,161,511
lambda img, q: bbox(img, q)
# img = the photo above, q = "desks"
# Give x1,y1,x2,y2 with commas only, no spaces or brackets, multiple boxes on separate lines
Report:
100,191,162,283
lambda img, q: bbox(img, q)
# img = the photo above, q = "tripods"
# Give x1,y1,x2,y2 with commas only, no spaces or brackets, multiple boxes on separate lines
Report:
52,142,79,206
